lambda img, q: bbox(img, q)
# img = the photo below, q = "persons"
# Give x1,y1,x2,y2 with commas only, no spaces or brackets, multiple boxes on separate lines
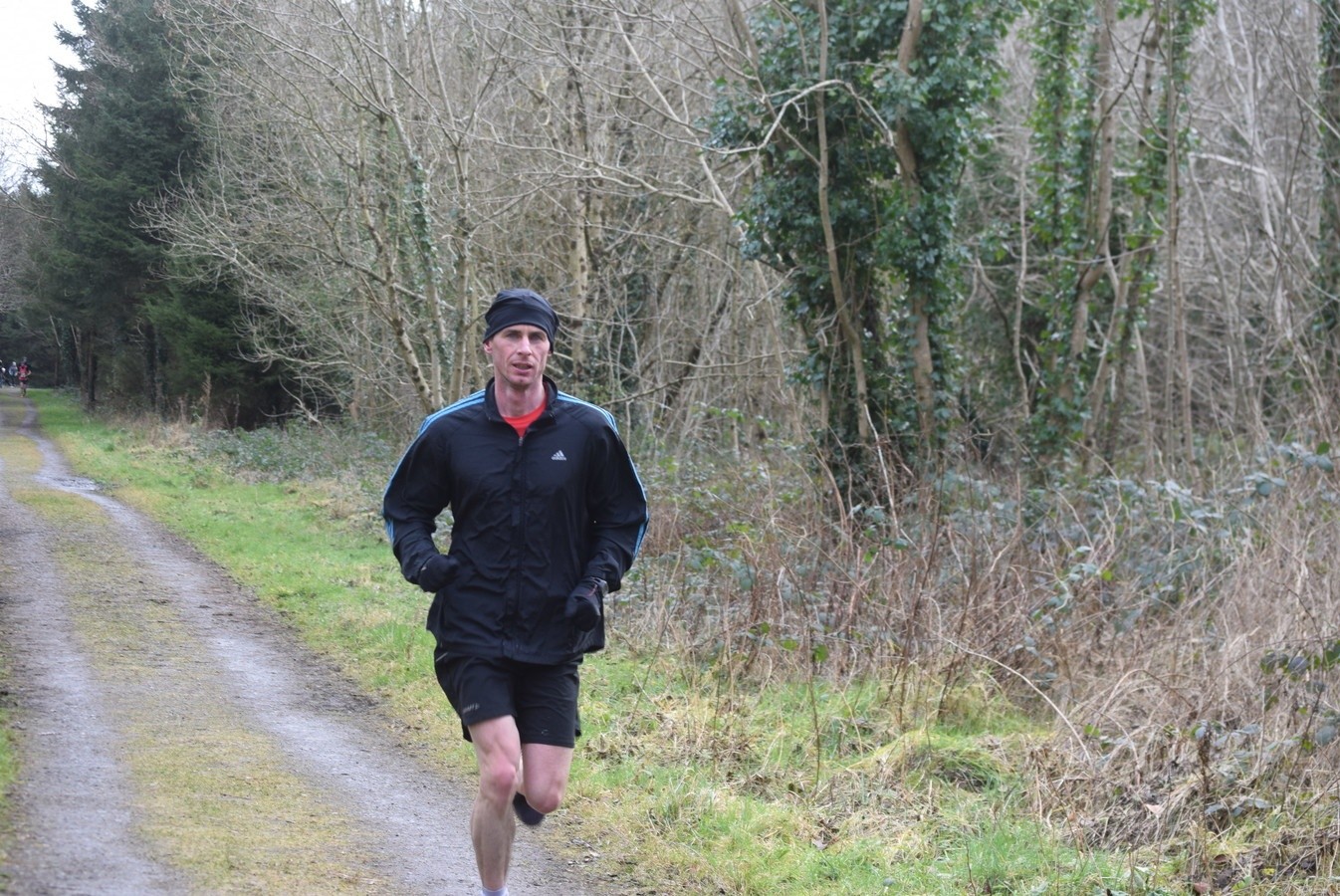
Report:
379,287,651,896
0,358,32,398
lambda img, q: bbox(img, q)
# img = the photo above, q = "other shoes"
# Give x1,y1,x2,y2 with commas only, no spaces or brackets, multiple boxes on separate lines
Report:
512,792,544,825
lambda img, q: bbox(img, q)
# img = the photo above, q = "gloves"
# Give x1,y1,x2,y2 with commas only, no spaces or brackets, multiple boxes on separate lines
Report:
417,554,459,594
566,580,606,633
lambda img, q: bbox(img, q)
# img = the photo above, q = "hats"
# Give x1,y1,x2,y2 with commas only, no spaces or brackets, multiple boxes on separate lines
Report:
483,291,558,352
